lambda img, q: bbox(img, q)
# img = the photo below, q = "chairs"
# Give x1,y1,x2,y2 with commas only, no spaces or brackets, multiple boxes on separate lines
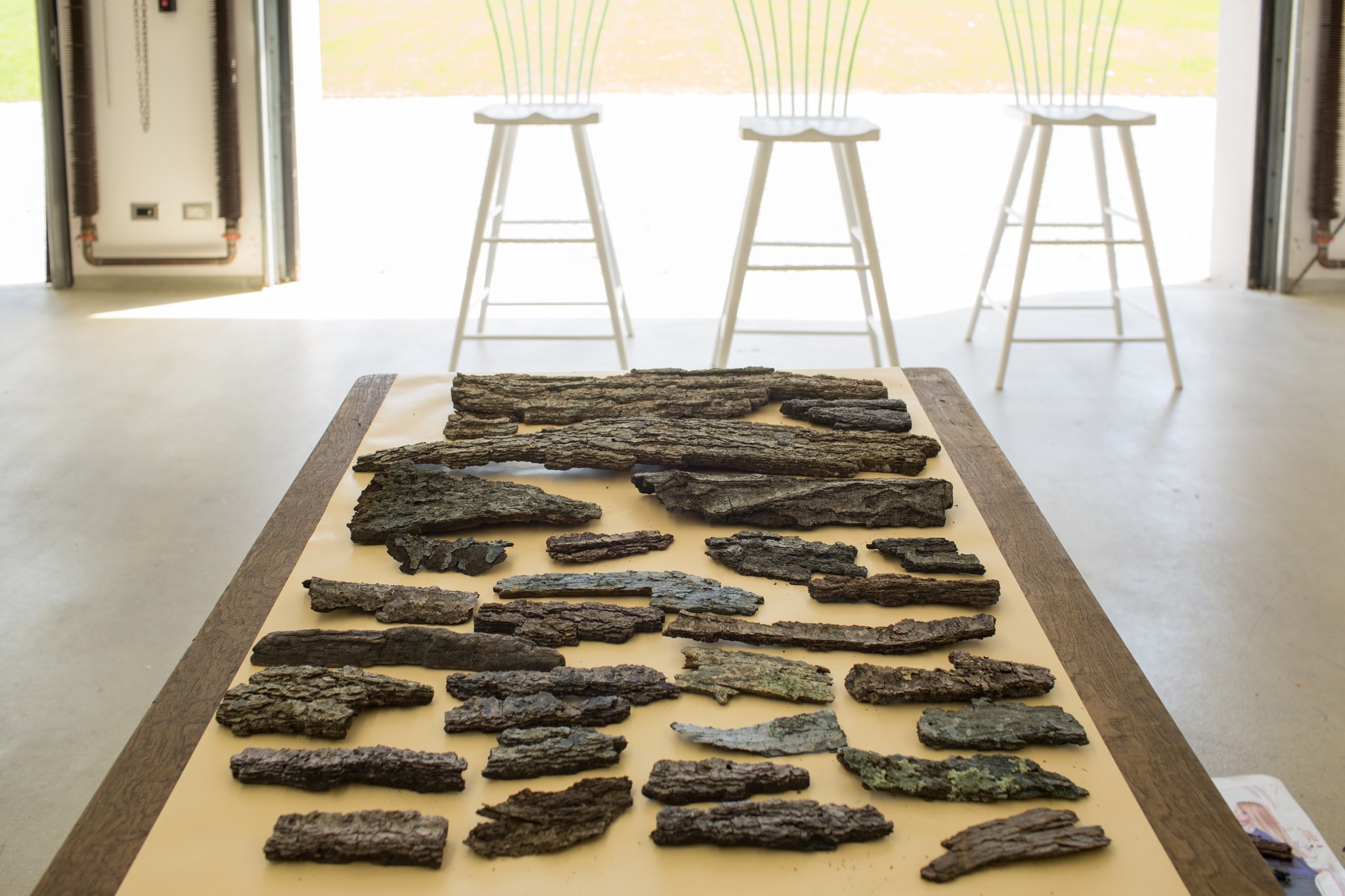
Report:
964,0,1185,390
709,0,901,371
448,0,637,376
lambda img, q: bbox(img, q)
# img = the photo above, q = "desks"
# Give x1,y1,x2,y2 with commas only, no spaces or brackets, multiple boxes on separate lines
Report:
31,366,1291,896
